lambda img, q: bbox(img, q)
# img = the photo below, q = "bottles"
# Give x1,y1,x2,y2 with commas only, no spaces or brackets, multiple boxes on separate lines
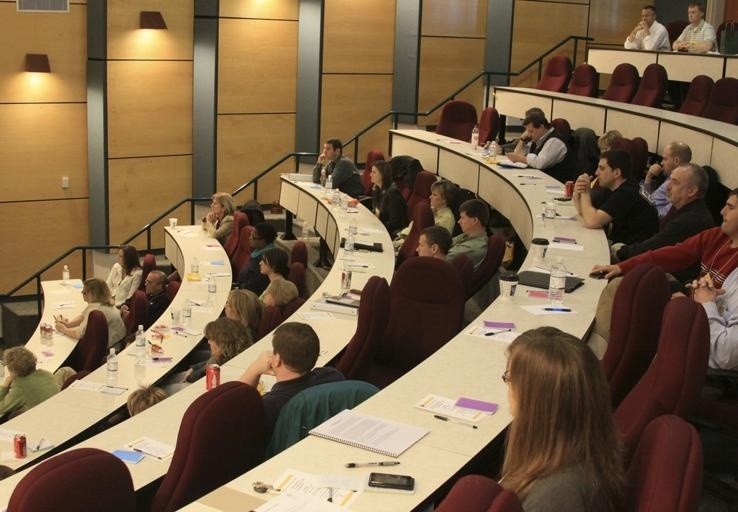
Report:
470,124,480,149
134,323,146,363
107,348,119,384
191,256,200,278
63,266,70,283
184,302,192,317
547,256,567,303
323,174,359,257
483,140,497,162
207,277,216,300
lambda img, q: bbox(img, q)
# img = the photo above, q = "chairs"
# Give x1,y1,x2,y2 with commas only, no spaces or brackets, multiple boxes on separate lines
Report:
440,99,729,512
537,51,736,123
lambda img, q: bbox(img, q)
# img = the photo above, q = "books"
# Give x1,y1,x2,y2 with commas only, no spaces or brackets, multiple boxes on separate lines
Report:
309,409,428,457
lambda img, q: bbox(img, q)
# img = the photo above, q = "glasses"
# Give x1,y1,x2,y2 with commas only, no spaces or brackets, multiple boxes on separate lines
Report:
502,369,511,383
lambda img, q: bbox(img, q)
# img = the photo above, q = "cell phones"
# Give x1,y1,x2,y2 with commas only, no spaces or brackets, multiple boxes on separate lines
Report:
368,473,413,489
591,272,606,280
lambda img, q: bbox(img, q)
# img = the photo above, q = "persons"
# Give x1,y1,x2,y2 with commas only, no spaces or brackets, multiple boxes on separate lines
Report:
624,5,671,50
673,4,718,52
498,326,627,509
55,278,126,348
239,321,348,432
126,192,300,418
312,137,488,273
498,110,738,371
0,347,60,415
106,245,142,305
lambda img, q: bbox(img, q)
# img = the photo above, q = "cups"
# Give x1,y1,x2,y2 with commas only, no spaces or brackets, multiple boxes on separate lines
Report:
169,218,178,230
545,199,557,218
531,237,550,260
498,271,520,297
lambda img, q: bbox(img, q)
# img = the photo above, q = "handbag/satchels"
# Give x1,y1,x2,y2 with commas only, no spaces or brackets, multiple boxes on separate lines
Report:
720,22,738,54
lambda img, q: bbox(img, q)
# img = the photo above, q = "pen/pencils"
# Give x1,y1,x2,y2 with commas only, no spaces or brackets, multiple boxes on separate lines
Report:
133,448,162,460
345,461,400,468
542,308,572,312
434,415,477,429
107,385,128,390
349,264,368,268
484,328,512,336
520,183,536,185
552,240,577,244
153,358,174,360
176,331,187,337
37,438,44,450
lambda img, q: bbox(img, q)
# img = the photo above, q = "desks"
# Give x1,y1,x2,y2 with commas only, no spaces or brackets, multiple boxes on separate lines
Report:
2,127,615,510
494,85,738,211
